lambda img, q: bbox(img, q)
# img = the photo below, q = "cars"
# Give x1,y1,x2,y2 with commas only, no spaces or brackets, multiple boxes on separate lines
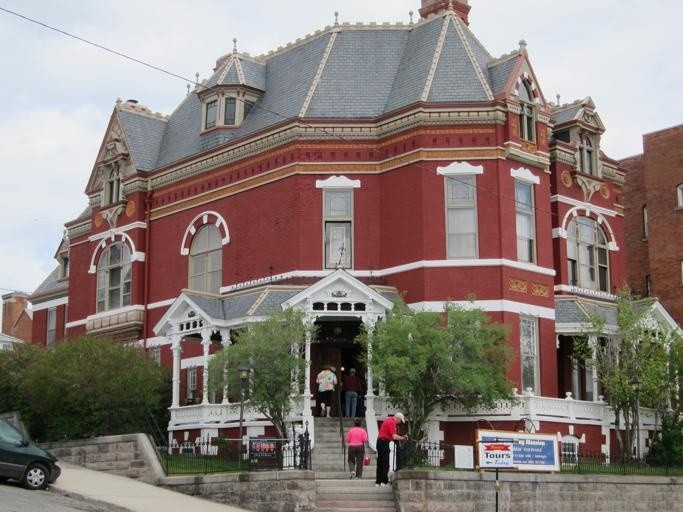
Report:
0,416,62,490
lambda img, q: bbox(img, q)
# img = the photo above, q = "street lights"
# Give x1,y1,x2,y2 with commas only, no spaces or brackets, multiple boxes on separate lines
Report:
237,363,251,470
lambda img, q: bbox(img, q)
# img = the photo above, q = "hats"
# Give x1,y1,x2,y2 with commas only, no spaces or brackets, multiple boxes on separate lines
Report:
395,412,405,424
349,368,356,372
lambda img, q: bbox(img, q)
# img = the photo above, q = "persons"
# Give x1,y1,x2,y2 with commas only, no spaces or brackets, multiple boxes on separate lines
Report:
374,411,409,486
341,367,362,417
343,418,369,479
315,365,337,417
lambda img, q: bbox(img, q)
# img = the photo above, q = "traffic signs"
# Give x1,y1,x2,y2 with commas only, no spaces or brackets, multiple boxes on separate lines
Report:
477,440,513,468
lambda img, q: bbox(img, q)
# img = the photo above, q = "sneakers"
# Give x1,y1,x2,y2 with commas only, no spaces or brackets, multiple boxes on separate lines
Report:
375,483,386,488
350,472,361,480
320,410,325,416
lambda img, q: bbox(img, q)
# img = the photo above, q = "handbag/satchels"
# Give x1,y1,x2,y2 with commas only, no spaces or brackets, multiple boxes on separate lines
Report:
364,456,371,465
